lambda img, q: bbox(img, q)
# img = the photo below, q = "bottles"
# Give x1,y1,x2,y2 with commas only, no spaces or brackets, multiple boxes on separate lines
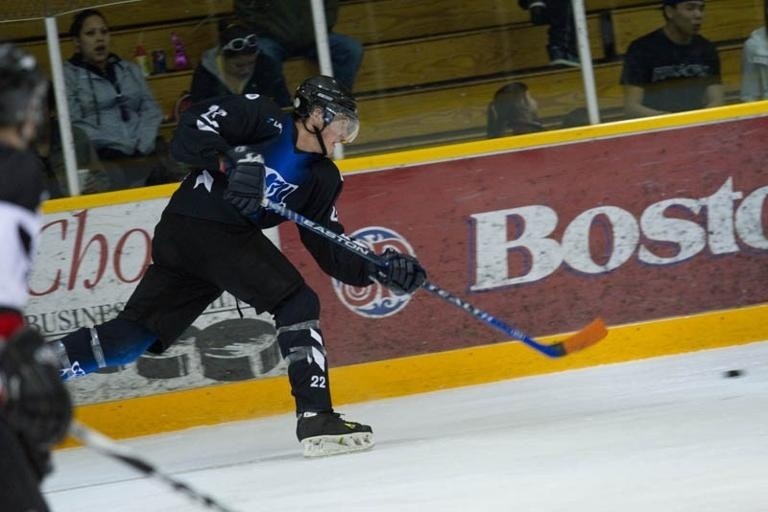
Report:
133,45,151,77
169,31,189,71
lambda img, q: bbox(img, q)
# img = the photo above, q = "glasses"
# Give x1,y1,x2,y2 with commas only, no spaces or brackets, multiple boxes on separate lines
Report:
223,34,258,51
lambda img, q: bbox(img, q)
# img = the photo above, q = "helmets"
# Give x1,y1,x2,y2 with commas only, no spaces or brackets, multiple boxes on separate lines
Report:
292,74,360,143
1,45,51,128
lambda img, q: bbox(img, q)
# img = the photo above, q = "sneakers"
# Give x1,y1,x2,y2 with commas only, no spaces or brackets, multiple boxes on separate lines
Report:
295,408,373,442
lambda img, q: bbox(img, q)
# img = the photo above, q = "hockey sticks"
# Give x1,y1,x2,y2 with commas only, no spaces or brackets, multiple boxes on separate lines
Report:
261,199,607,358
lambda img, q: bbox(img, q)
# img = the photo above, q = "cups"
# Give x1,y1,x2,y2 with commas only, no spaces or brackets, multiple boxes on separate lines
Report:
152,49,166,75
77,167,90,193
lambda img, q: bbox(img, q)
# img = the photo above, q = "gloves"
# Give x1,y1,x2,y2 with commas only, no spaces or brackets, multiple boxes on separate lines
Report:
224,163,265,215
369,247,426,296
1,327,71,447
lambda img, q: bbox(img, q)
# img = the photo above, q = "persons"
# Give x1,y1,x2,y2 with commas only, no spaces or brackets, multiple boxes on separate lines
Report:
232,0,367,91
484,81,590,138
33,72,427,443
516,0,579,68
739,0,768,102
61,8,170,160
190,18,293,108
617,0,725,120
0,42,77,512
